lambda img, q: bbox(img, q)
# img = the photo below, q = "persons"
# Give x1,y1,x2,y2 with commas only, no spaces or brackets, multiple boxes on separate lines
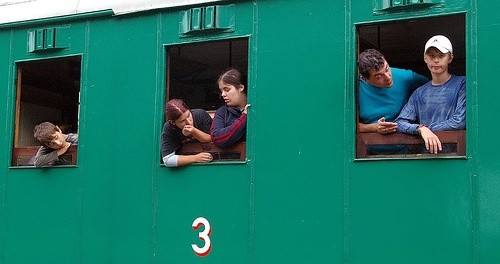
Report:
393,35,466,154
210,69,251,159
162,99,213,168
357,49,430,156
33,122,78,167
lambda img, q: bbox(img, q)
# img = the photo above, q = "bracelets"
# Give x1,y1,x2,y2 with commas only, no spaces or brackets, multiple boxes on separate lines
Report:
417,123,428,136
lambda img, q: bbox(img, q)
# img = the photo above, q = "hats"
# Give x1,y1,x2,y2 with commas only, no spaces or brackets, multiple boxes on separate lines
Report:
424,35,453,56
